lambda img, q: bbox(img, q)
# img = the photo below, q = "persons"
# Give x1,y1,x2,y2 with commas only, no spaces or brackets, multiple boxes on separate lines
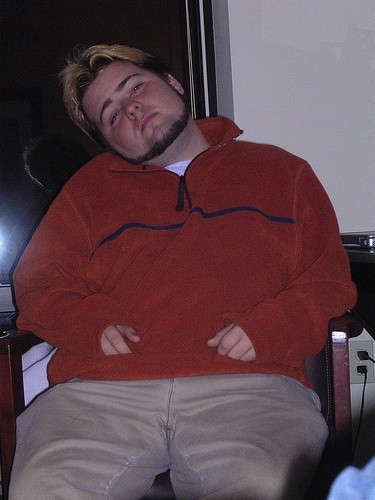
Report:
6,44,358,500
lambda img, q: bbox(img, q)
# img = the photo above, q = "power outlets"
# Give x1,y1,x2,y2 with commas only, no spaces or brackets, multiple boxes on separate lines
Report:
349,340,375,384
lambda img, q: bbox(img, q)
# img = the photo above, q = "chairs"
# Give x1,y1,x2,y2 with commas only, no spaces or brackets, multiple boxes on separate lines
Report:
0,316,355,500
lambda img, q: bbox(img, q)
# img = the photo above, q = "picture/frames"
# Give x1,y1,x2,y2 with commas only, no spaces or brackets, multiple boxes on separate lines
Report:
0,85,48,212
